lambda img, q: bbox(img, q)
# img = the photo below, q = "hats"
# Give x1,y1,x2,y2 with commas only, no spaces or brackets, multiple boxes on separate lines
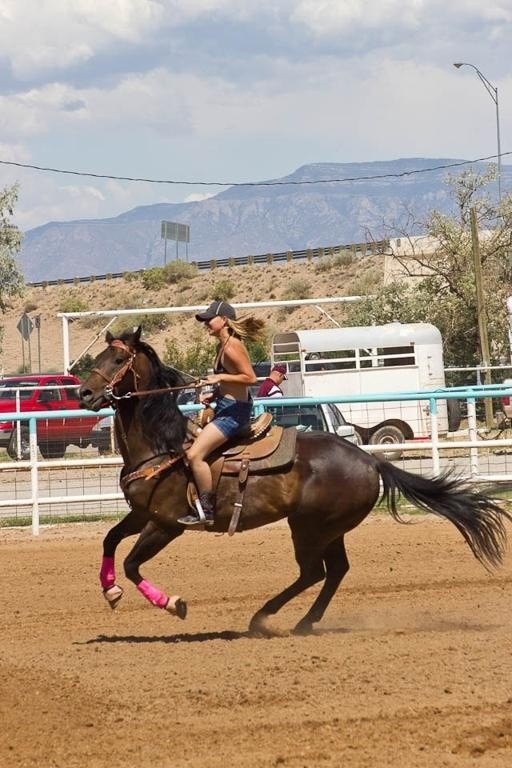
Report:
271,363,288,380
195,301,236,322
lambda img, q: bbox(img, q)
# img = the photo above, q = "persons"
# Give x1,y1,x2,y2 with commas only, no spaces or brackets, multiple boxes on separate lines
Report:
176,300,257,529
257,364,288,398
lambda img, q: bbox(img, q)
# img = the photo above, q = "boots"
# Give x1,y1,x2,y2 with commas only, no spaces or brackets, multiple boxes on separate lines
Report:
177,492,216,525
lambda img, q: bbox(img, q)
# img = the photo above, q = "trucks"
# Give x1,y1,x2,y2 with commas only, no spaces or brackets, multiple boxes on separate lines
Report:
268,322,461,460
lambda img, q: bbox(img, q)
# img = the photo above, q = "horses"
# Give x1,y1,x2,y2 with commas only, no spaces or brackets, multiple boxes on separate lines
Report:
73,320,512,635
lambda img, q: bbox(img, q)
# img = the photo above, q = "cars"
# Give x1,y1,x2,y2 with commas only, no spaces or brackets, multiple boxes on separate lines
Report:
253,397,363,448
0,374,110,461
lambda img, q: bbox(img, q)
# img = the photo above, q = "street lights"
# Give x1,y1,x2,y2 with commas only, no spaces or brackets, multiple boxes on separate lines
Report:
454,62,502,203
